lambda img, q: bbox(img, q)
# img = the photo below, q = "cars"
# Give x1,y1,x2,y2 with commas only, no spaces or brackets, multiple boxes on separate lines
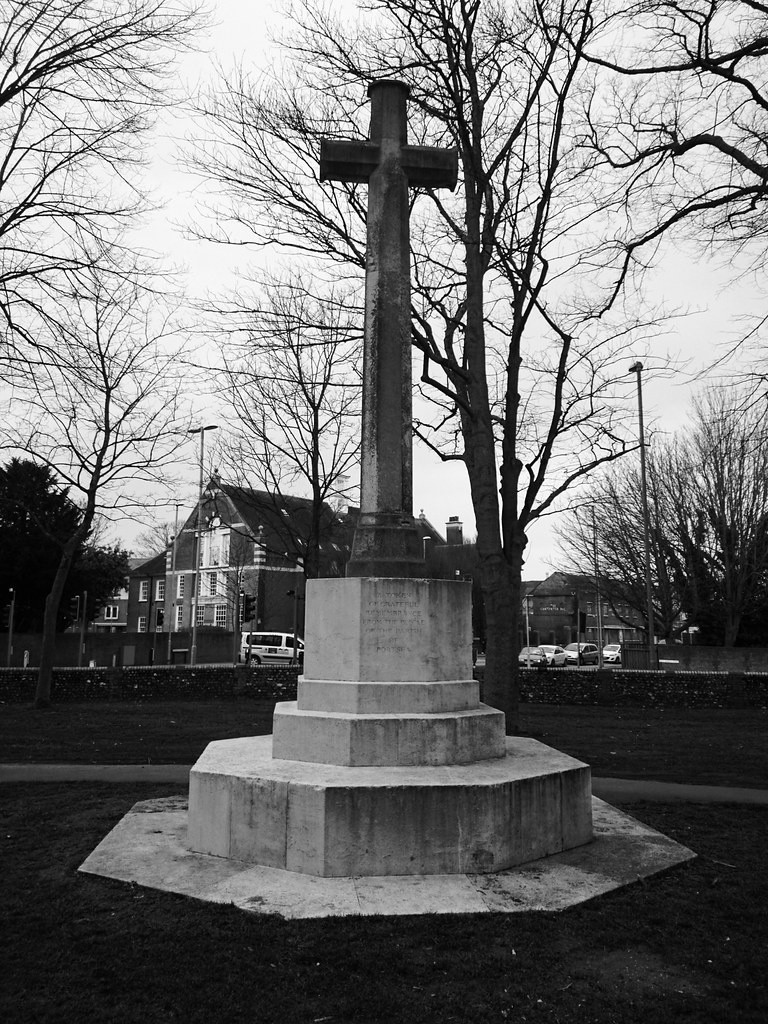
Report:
518,647,547,667
537,645,568,667
603,645,621,664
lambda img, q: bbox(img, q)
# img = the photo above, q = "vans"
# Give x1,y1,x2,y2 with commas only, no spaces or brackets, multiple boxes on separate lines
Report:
240,631,305,666
564,643,600,665
